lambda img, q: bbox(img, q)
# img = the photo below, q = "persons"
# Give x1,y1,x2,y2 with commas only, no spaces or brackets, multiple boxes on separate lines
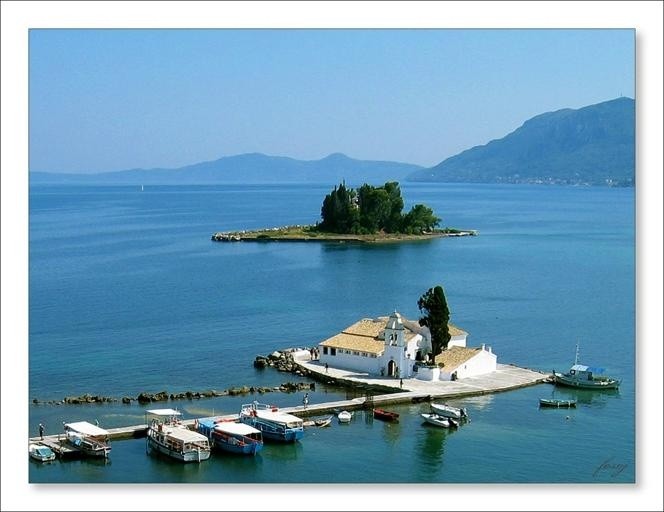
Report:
400,378,403,388
310,348,318,360
63,422,68,434
303,393,309,409
325,363,328,372
95,419,99,427
39,424,44,440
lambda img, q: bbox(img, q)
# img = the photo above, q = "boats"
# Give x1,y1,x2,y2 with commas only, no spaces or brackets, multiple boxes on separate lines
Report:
238,402,303,443
430,403,465,418
145,415,210,463
198,418,262,455
371,408,398,420
420,413,450,428
552,365,622,390
539,398,577,407
337,411,350,422
63,420,111,458
29,445,55,462
